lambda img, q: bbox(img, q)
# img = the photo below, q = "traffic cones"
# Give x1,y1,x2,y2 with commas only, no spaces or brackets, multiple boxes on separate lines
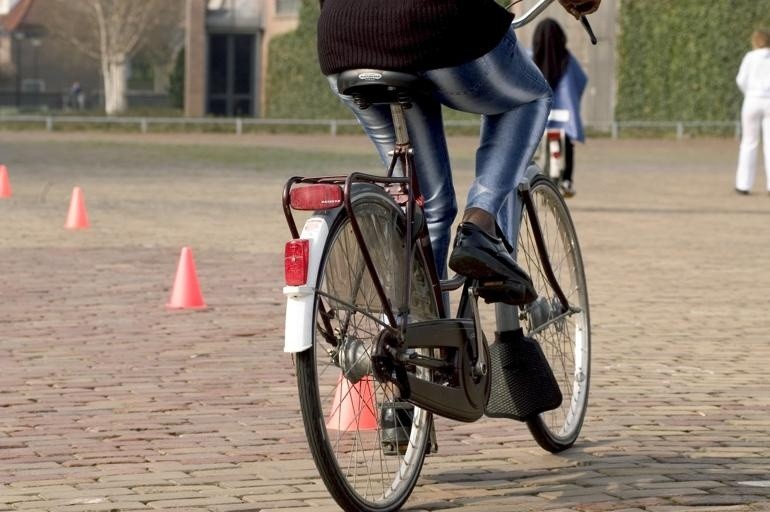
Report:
1,164,11,198
65,187,89,230
166,246,206,310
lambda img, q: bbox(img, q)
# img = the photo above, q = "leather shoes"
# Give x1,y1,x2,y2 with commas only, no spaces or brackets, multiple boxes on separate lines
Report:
451,220,538,305
381,397,412,445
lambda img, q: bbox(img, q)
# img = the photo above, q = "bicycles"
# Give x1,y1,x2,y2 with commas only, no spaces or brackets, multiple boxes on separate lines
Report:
281,1,598,512
543,127,566,190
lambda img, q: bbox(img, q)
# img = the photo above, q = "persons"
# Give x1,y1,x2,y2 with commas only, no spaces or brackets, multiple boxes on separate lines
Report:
734,31,769,194
526,18,588,195
312,2,598,454
